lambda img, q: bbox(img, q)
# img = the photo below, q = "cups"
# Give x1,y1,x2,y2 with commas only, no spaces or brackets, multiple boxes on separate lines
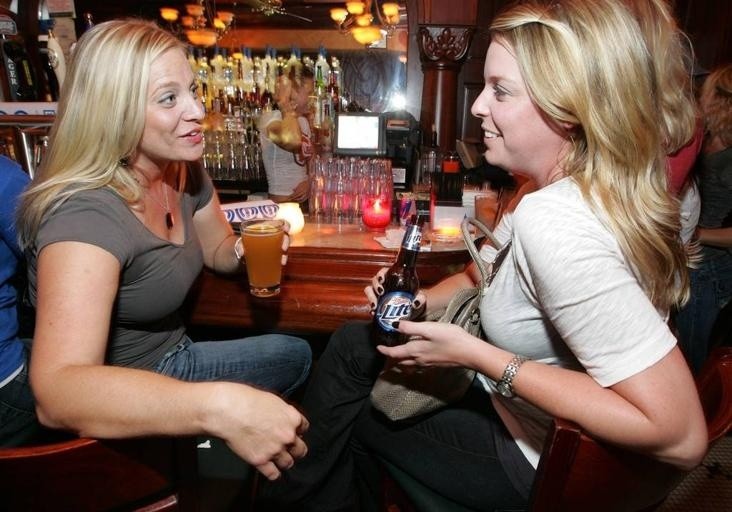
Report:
444,154,461,173
474,194,498,236
183,44,351,128
361,197,393,232
239,220,285,297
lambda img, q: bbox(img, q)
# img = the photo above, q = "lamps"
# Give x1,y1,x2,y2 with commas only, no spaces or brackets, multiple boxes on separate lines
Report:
331,0,401,46
160,0,234,47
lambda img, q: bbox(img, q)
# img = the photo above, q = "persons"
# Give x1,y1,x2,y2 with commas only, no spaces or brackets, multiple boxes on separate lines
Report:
1,18,314,512
269,1,732,511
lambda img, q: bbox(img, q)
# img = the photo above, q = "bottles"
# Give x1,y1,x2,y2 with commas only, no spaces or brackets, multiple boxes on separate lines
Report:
320,103,334,152
311,155,394,222
422,131,443,188
200,116,267,182
371,212,426,349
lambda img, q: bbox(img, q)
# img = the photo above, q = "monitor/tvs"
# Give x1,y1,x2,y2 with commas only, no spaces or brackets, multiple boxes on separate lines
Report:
333,111,388,157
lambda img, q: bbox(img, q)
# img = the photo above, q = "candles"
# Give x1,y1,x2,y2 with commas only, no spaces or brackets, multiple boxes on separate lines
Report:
364,199,390,228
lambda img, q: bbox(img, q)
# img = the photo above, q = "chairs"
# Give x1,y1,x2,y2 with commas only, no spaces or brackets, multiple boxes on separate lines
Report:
527,344,732,512
0,431,186,511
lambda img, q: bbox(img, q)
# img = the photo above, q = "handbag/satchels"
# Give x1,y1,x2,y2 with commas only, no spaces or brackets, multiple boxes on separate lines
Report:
369,216,503,421
